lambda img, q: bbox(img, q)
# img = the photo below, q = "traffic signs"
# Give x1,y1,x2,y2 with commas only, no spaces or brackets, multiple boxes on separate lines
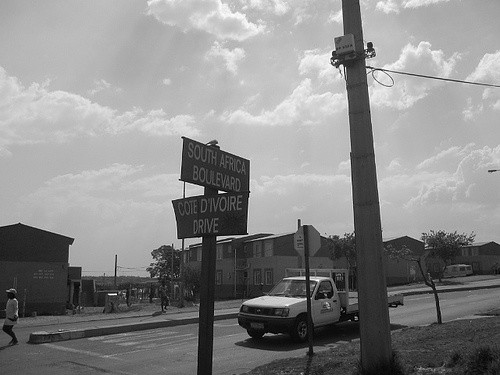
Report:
171,135,251,239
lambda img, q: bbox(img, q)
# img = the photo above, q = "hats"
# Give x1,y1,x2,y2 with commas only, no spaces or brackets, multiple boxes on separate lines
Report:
6,288,17,297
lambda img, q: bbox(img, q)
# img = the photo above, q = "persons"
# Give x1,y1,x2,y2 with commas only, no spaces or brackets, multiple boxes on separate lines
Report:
2,288,19,346
159,279,169,313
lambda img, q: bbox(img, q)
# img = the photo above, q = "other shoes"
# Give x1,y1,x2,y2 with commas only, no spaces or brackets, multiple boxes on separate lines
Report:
164,307,168,310
162,310,166,313
9,339,18,345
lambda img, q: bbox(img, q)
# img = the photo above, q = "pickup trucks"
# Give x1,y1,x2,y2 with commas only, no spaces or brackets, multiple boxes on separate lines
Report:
237,268,404,341
